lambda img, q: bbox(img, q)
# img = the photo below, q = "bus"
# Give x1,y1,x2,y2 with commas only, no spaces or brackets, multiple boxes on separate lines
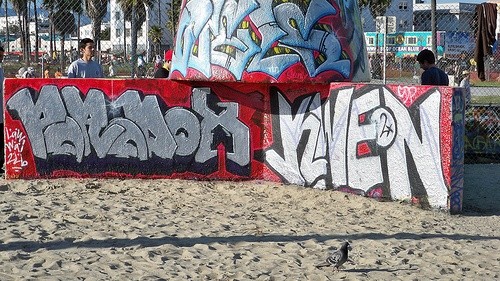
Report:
362,31,448,58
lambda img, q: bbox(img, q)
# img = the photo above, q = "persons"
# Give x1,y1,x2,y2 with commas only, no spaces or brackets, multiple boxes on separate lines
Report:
370,54,382,80
388,55,415,72
155,50,173,78
68,38,103,78
457,50,471,103
417,49,449,86
7,62,63,79
138,55,163,77
108,55,118,77
43,46,77,63
0,46,5,173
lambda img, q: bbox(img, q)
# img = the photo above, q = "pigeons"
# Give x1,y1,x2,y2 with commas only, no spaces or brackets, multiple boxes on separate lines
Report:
316,241,350,272
